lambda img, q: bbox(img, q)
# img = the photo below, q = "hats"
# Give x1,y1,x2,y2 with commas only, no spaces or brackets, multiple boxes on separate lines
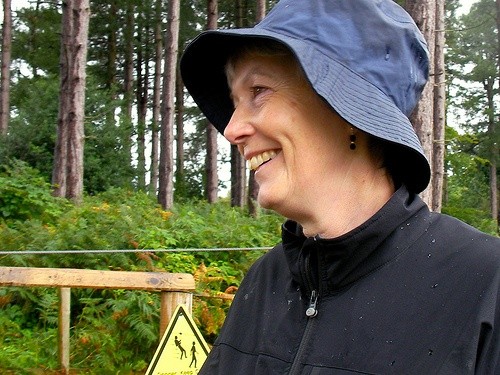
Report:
181,0,431,196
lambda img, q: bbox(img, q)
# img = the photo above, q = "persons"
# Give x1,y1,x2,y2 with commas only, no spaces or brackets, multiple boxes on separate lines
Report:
181,0,500,374
